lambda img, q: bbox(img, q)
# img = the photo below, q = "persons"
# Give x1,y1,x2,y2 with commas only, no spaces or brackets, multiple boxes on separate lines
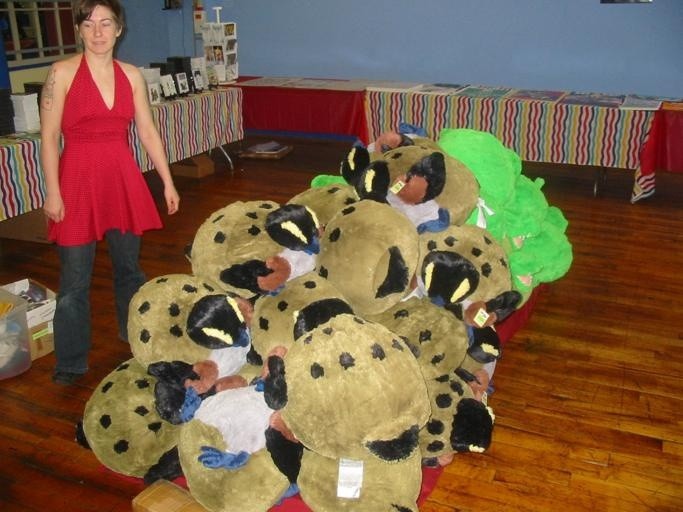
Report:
40,0,180,383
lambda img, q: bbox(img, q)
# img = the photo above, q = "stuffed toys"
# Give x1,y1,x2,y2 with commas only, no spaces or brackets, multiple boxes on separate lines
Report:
76,125,573,512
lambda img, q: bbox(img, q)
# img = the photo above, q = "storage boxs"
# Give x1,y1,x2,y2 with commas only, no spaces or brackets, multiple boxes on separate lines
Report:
0,208,55,244
0,277,60,380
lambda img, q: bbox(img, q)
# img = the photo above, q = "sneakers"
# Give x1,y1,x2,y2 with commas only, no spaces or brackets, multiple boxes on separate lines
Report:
53,371,80,388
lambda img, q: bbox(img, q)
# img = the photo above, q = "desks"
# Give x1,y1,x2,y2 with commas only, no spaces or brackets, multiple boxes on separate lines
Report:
228,77,683,198
0,85,242,224
